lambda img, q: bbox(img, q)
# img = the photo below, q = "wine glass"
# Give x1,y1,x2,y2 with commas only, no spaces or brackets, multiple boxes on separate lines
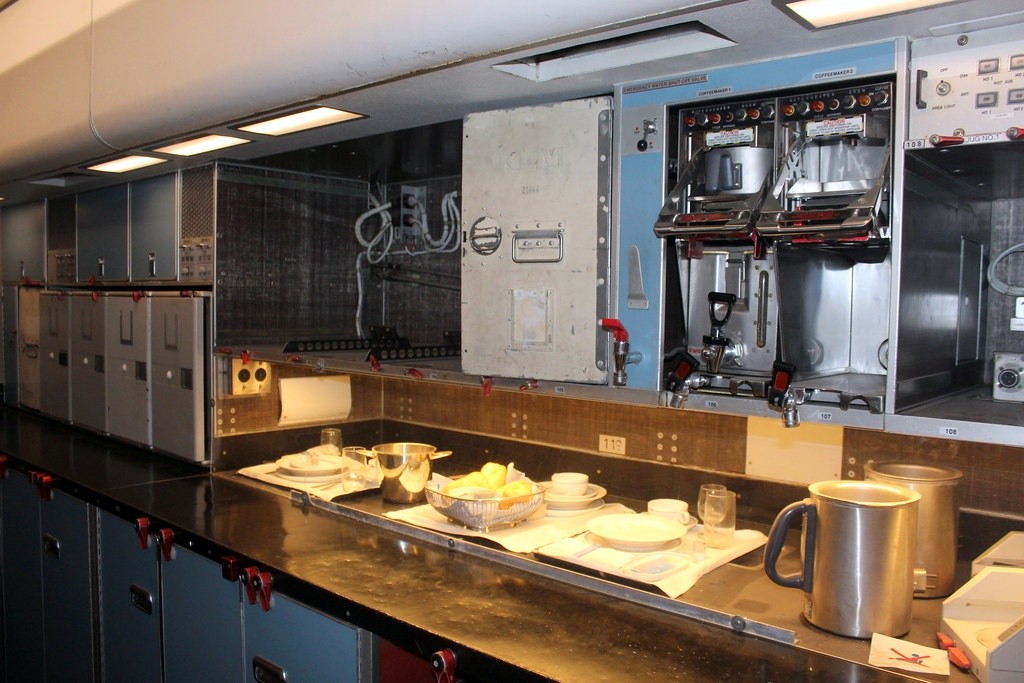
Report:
697,483,728,541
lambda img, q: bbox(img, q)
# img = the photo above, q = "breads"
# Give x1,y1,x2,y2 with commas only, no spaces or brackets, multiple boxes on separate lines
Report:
443,462,531,510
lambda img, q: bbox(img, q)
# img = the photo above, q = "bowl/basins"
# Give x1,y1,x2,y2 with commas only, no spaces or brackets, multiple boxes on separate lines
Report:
281,454,312,469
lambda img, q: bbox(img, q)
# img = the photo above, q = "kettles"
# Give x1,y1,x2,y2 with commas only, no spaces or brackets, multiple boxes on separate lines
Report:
763,480,921,638
864,460,964,598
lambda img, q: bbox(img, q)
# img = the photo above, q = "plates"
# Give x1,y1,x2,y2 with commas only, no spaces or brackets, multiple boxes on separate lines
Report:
587,514,688,551
275,452,352,483
639,511,698,530
537,482,607,517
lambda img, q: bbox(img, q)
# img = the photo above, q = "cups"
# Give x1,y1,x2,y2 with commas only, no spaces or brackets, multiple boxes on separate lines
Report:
551,472,589,496
341,446,379,492
321,428,342,468
647,499,690,524
704,490,736,549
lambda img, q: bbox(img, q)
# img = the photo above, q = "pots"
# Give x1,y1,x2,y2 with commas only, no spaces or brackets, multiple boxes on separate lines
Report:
357,442,453,504
